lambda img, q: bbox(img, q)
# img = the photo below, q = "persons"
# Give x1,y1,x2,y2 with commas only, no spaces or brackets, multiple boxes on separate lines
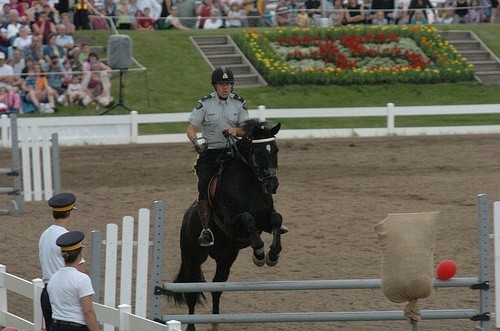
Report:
304,0,500,27
186,67,289,245
46,230,99,331
101,0,308,31
0,0,114,114
39,192,85,331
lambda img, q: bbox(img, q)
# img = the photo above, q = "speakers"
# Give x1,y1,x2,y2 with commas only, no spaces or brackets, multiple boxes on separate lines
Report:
108,35,132,70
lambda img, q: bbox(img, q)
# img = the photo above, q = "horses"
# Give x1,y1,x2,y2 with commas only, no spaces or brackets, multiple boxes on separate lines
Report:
166,120,284,331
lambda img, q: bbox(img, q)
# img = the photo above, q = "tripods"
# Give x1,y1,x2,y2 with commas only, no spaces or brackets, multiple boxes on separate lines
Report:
99,69,132,115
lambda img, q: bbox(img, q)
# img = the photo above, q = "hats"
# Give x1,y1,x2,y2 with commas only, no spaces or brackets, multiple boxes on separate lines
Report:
56,231,86,251
212,67,234,84
48,193,77,211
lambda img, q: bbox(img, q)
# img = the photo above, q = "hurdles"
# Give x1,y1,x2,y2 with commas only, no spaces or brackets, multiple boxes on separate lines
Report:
88,194,500,331
0,114,62,216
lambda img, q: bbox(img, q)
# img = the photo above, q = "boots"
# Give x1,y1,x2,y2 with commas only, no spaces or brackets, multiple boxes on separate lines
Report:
197,199,212,244
280,225,288,234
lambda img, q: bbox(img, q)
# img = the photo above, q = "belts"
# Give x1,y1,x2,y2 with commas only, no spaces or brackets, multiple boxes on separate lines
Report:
52,320,81,327
